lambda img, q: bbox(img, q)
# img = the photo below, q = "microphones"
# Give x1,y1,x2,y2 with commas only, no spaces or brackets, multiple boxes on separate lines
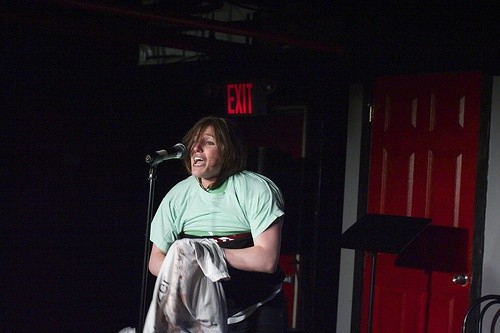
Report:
146,142,188,167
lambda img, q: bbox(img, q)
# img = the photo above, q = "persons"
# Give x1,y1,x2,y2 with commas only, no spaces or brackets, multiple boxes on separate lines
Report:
146,114,289,332
